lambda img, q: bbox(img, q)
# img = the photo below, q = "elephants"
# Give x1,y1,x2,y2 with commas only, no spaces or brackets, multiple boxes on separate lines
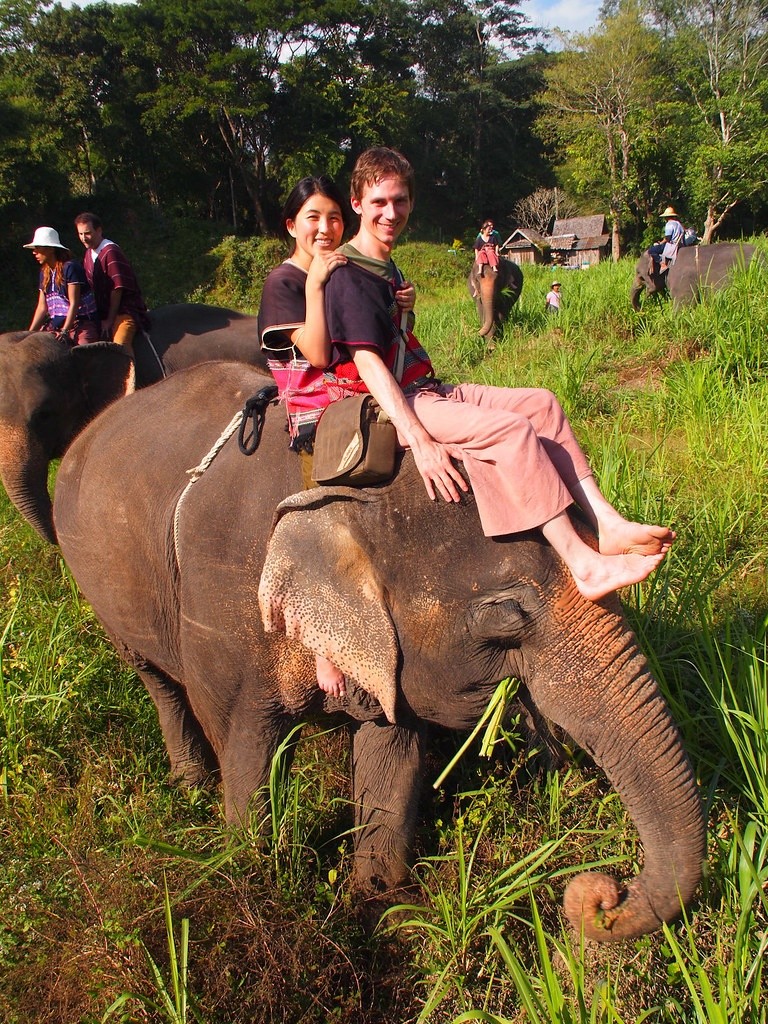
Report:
630,243,764,312
0,302,707,942
468,257,523,351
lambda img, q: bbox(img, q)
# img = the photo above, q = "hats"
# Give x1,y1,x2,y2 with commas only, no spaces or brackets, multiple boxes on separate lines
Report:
658,206,678,217
22,226,69,251
551,280,561,287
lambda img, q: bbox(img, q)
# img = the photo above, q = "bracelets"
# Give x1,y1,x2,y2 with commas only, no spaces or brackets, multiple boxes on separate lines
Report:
62,329,69,336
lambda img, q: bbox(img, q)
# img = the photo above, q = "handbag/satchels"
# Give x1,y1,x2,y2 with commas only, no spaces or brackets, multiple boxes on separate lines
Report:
310,267,409,487
661,231,684,265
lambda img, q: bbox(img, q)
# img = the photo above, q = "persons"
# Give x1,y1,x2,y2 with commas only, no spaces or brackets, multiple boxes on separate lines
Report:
75,213,146,364
648,207,685,275
546,281,562,315
256,148,678,600
23,226,99,345
472,220,503,275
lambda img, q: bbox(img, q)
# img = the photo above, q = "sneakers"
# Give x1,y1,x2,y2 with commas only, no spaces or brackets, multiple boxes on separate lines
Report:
659,267,669,275
